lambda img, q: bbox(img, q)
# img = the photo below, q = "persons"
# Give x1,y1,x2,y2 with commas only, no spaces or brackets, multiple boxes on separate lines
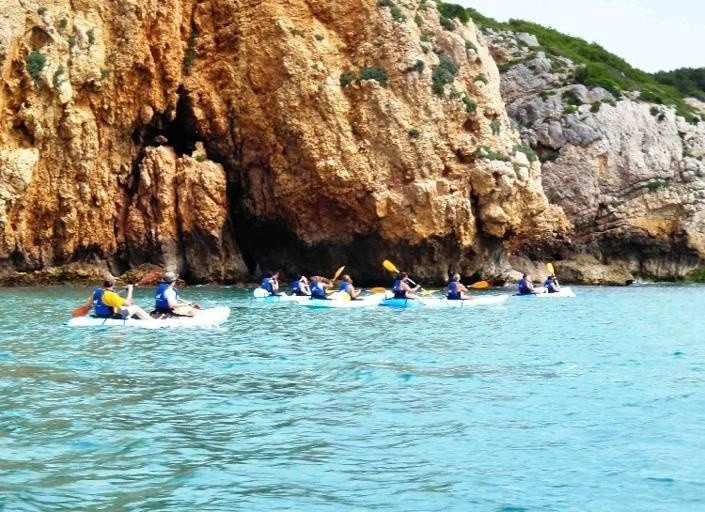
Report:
261,271,290,296
155,271,199,316
519,273,537,294
445,274,473,299
307,274,335,299
292,273,311,295
340,274,364,301
85,273,153,320
391,272,421,299
545,273,560,292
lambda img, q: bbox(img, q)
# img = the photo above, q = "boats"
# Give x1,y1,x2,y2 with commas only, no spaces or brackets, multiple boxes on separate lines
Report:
256,290,509,309
512,287,575,298
61,305,231,330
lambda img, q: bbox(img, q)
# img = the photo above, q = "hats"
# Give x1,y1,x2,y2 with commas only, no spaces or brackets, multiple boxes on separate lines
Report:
163,271,177,282
103,274,116,289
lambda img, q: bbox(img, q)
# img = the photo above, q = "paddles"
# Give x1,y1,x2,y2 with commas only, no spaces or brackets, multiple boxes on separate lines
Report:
382,260,434,297
547,263,560,292
72,272,159,317
330,266,346,282
253,288,275,298
326,287,386,294
419,281,488,297
